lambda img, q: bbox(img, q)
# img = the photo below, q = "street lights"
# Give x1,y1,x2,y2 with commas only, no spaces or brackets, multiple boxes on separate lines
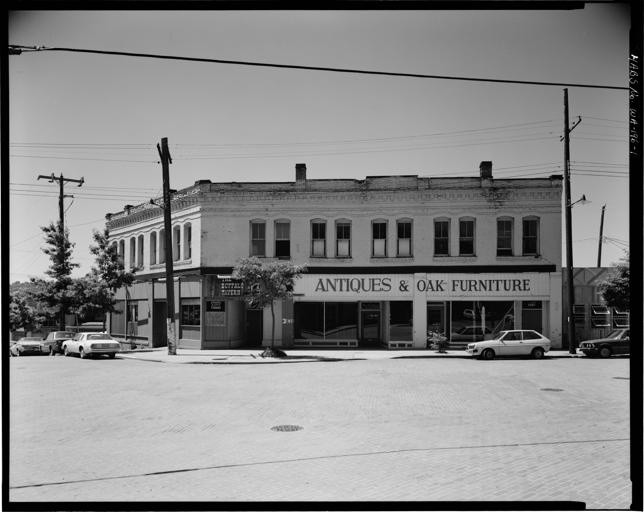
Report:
563,191,592,359
149,198,181,356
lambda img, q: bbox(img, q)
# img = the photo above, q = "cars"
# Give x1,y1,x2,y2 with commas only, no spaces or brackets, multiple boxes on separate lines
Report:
10,335,49,359
463,308,474,320
452,326,495,341
39,330,76,354
60,332,121,359
578,326,632,358
461,329,552,360
